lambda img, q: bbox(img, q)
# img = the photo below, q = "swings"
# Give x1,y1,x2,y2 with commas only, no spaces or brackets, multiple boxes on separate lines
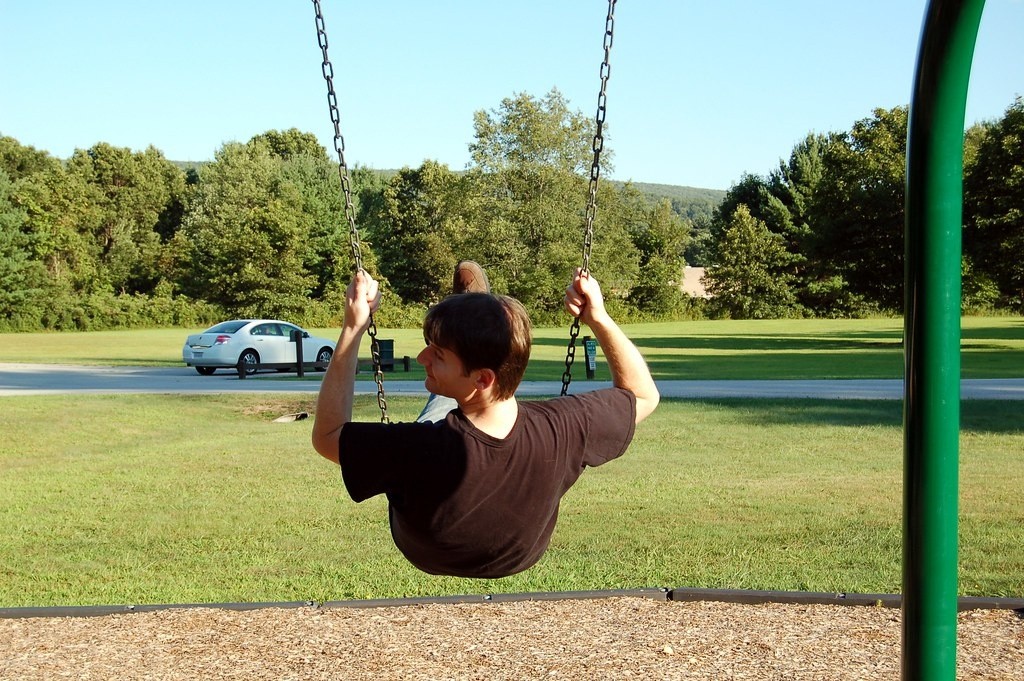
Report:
313,0,618,463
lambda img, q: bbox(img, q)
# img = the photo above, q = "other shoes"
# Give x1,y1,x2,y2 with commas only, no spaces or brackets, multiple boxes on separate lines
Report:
452,259,491,295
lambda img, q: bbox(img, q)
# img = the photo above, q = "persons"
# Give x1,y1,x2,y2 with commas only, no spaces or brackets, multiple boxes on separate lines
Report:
261,326,272,335
312,260,660,579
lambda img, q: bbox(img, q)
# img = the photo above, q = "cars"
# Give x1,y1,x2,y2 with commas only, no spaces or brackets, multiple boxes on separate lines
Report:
182,319,336,375
